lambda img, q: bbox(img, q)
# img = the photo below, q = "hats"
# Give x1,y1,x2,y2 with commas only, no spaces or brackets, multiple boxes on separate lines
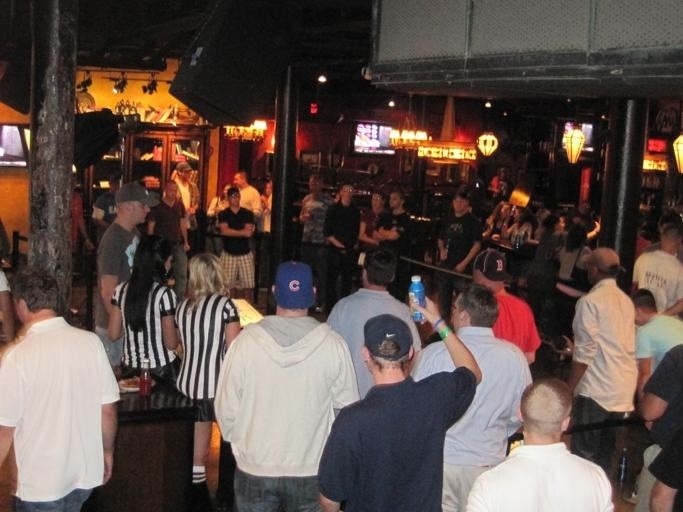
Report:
273,261,316,310
115,181,160,208
176,162,192,171
364,313,413,361
581,247,626,274
474,248,507,281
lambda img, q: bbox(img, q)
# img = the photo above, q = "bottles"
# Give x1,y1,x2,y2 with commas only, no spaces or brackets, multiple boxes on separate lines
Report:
139,359,151,397
617,448,629,483
410,276,425,320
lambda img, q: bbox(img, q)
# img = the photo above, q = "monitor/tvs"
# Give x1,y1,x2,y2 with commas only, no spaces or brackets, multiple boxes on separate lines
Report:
555,117,608,159
349,120,397,158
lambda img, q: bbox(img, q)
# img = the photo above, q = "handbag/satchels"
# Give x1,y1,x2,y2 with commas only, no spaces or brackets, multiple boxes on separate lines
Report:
571,245,587,280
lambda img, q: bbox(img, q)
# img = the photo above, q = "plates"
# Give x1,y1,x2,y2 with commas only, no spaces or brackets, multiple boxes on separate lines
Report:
118,378,155,392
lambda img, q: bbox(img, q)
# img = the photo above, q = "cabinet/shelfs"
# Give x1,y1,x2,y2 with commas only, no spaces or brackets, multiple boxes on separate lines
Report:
128,122,212,216
75,127,128,275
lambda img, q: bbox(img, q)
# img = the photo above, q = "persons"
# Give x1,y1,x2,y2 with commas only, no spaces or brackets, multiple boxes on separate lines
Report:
557,223,592,338
69,173,93,314
174,163,201,232
637,342,683,512
565,246,636,512
0,222,15,345
631,288,683,395
260,181,274,291
146,178,191,302
470,247,541,366
93,180,158,371
299,173,330,318
234,171,263,222
488,200,551,255
317,296,482,512
465,378,615,512
213,259,360,512
207,183,231,216
631,223,683,318
359,188,388,249
321,247,421,400
409,283,534,511
371,188,413,261
0,267,121,512
526,213,601,349
323,182,361,320
214,186,257,305
174,253,241,481
434,190,482,323
107,232,183,383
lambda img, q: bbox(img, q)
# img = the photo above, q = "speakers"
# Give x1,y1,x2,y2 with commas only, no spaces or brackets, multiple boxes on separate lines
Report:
169,0,295,127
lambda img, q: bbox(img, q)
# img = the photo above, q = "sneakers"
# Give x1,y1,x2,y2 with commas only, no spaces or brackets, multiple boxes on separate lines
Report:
623,488,638,504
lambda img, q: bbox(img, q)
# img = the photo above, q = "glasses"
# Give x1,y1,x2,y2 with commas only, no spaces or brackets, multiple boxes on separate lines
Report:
344,188,353,192
373,196,380,200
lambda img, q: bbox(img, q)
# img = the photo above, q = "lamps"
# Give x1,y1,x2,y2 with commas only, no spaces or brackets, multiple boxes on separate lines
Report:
388,94,432,153
671,103,682,175
563,100,584,165
476,100,498,158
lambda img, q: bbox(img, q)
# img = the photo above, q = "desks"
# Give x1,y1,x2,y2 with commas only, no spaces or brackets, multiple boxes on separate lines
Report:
114,371,201,512
